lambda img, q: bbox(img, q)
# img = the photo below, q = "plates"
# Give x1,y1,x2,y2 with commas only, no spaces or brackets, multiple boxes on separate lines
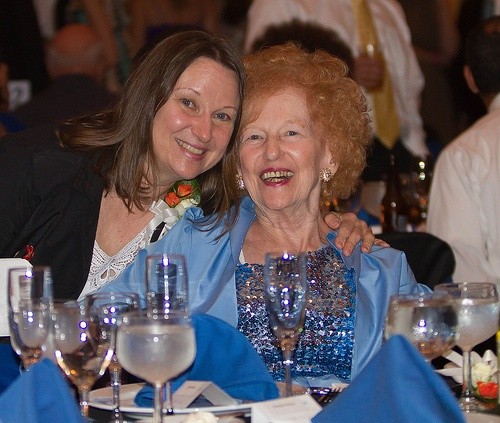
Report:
87,382,311,420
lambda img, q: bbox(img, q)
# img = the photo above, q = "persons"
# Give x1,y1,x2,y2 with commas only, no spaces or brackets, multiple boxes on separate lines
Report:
0,30,390,348
83,44,440,386
214,0,500,309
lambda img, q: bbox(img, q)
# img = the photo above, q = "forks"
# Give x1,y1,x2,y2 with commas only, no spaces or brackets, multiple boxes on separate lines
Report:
316,387,344,406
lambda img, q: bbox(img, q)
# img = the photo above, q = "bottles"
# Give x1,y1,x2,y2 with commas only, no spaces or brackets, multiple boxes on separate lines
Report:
383,152,407,233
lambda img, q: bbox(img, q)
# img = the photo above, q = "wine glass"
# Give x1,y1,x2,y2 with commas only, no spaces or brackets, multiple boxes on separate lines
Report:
433,283,500,413
115,311,196,423
264,251,310,398
398,159,432,232
7,266,54,369
82,293,143,423
51,308,115,418
146,254,190,416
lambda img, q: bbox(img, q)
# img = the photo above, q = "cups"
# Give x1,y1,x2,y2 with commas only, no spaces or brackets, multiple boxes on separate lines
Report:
360,43,384,92
383,293,459,362
